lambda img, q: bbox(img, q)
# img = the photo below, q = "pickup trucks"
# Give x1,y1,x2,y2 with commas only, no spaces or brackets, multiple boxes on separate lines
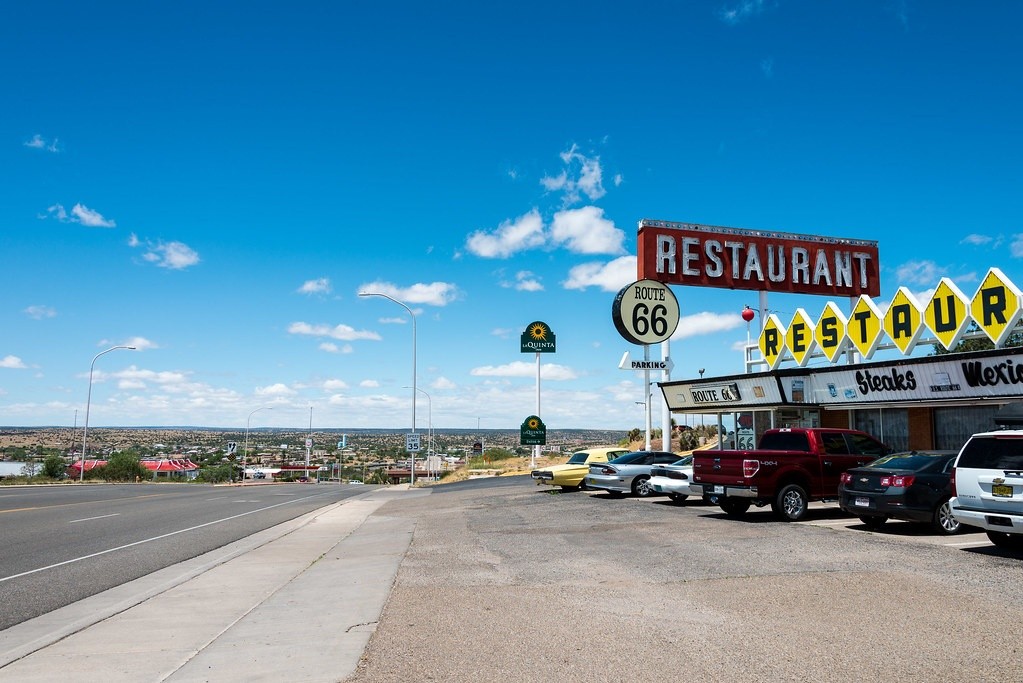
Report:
689,427,897,522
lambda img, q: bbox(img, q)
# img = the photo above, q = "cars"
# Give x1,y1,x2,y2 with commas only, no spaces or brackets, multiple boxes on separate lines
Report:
646,456,719,506
531,448,633,491
585,451,685,497
253,472,266,479
838,449,965,535
300,476,307,482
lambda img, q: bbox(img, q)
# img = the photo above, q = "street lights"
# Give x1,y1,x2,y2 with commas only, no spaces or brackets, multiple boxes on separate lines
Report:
416,418,438,481
80,345,136,481
358,292,420,487
402,386,431,481
242,407,272,484
305,432,323,477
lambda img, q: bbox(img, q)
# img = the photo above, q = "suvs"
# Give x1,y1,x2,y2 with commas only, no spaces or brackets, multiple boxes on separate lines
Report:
948,429,1023,547
